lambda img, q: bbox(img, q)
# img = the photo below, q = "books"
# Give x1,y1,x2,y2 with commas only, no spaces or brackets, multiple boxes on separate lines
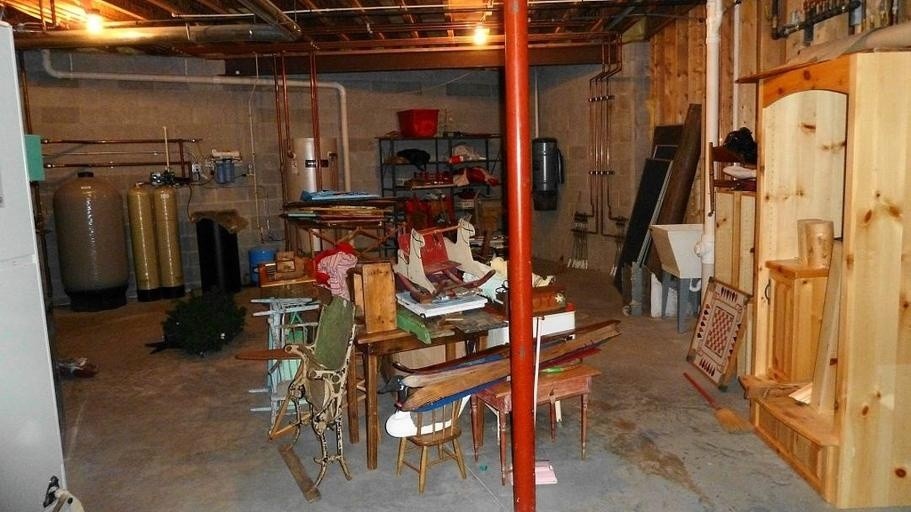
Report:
511,459,558,485
397,302,486,318
396,286,489,312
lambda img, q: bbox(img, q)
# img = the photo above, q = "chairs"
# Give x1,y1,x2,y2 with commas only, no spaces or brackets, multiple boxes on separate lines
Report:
268,293,360,499
395,387,467,493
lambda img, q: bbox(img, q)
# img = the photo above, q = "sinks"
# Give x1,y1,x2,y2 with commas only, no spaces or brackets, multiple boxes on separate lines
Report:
648,223,703,279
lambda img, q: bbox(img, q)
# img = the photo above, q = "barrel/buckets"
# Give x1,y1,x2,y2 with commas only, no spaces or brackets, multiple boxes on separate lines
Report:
248,245,279,285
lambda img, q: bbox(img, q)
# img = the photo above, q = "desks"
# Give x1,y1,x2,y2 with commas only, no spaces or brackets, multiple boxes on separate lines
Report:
347,303,508,469
469,365,603,486
283,217,390,274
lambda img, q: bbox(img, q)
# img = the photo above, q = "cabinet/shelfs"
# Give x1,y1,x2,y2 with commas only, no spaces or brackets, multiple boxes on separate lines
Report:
374,135,504,258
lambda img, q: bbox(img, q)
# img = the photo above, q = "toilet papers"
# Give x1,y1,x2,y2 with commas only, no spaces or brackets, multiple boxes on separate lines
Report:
796,218,821,267
806,220,835,271
